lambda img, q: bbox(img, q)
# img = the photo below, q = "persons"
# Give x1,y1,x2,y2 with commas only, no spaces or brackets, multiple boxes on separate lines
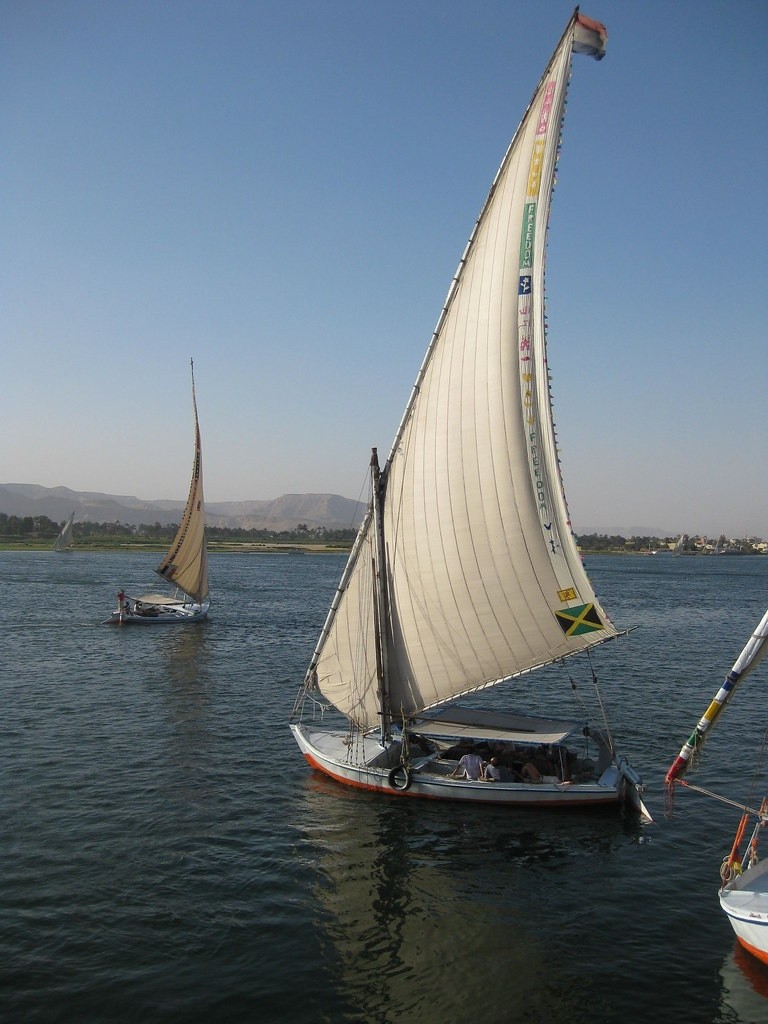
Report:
524,745,559,777
521,753,542,784
134,602,141,612
123,602,130,615
485,752,515,782
473,742,494,761
452,748,484,781
117,590,124,611
396,718,434,756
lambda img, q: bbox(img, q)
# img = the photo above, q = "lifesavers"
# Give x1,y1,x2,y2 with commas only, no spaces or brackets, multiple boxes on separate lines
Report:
386,766,415,793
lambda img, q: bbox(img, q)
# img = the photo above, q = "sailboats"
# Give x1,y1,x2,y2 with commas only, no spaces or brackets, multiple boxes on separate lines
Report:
107,355,211,625
50,510,77,554
294,9,646,816
